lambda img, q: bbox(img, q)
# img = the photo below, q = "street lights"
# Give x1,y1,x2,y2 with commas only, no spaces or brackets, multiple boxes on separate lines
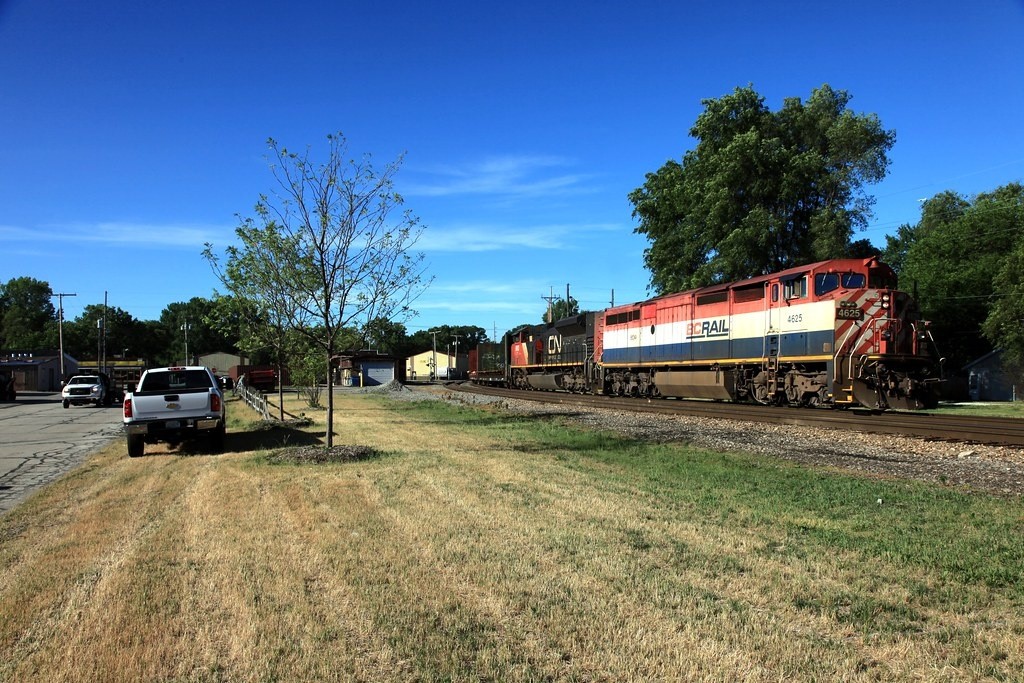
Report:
429,330,441,380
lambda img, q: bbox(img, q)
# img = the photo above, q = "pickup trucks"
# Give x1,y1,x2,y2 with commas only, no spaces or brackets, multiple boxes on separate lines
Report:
60,374,112,408
121,363,228,458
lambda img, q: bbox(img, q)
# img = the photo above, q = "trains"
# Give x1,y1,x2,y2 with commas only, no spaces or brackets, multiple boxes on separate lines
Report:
468,252,951,416
